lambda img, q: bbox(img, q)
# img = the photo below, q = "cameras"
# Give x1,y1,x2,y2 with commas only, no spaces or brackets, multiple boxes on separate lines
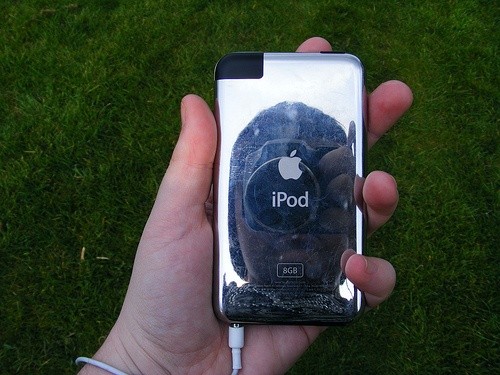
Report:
242,138,347,237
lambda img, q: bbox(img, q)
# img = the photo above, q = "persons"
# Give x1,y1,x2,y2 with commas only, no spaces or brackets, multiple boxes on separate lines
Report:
71,36,415,375
218,100,359,318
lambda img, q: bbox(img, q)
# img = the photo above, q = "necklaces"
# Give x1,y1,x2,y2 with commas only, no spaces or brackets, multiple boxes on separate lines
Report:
226,282,354,315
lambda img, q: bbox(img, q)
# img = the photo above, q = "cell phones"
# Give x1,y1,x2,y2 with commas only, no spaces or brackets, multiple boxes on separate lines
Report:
213,52,368,327
